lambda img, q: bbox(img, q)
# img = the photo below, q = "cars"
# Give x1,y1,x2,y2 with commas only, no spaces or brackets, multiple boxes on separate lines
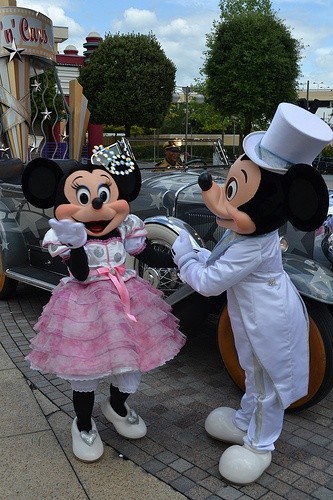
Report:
0,138,333,413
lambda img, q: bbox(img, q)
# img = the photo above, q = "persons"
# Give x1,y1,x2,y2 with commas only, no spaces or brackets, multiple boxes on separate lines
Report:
155,139,189,169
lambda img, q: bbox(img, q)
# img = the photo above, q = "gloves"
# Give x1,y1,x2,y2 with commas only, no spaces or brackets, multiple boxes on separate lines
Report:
197,248,212,267
123,214,147,257
48,218,87,249
171,229,199,269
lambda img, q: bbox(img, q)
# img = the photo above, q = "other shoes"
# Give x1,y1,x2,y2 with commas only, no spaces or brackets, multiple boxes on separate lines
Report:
218,445,272,485
101,394,146,439
71,416,104,462
204,407,248,445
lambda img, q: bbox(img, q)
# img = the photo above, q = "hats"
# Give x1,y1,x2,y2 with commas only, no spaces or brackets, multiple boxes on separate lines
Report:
242,102,333,175
163,140,183,151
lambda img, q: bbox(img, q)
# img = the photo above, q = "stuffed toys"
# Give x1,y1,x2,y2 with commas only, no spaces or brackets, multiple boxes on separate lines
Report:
170,102,333,486
22,145,186,462
